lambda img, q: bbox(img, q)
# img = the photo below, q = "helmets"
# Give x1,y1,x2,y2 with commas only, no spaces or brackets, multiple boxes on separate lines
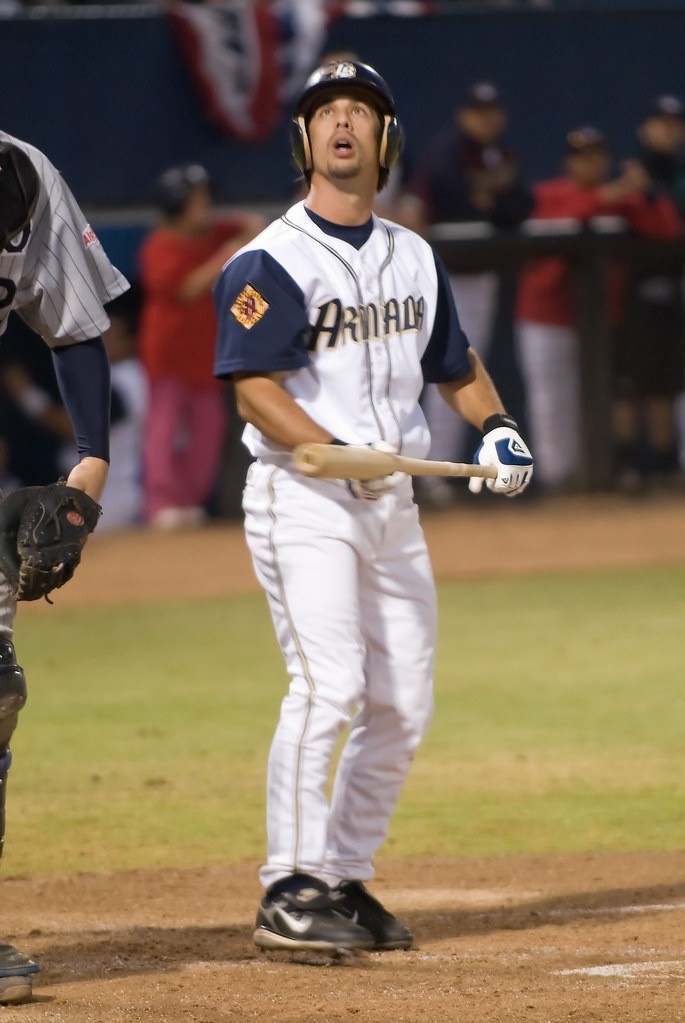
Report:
290,58,402,194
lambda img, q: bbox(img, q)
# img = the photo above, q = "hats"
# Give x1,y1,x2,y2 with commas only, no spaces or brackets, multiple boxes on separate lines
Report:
463,84,501,111
157,165,206,218
569,126,601,155
650,95,683,117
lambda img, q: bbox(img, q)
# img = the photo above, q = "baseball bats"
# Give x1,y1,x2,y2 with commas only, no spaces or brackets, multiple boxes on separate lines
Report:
291,441,498,479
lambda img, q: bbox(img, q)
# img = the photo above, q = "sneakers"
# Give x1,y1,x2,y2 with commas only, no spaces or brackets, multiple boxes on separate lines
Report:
252,871,374,955
330,878,413,949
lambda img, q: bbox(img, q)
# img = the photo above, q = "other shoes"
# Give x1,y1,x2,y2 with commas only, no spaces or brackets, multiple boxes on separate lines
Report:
0,975,33,1003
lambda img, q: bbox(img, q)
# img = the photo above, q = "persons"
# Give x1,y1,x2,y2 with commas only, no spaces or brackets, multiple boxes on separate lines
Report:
514,125,681,495
599,95,685,495
213,61,534,949
1,302,150,534
0,125,130,1006
399,80,539,503
140,158,261,528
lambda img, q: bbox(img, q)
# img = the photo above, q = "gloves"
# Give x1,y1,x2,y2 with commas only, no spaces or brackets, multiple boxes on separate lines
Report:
468,413,535,497
330,436,409,499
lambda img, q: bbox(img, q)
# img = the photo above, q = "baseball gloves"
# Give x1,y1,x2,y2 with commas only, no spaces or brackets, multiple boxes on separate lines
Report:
0,475,105,606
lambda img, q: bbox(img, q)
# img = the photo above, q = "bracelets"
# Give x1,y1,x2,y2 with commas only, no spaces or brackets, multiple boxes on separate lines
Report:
17,385,45,412
